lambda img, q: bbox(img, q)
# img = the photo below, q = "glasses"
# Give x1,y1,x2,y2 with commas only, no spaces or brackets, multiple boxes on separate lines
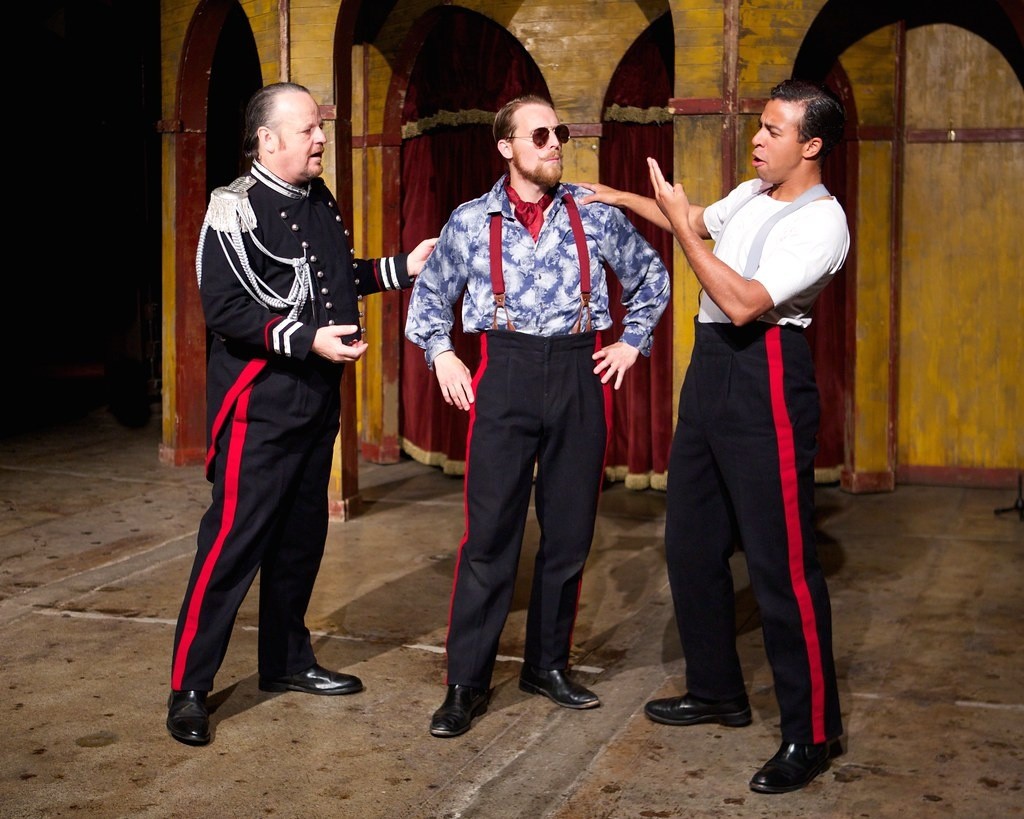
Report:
505,125,570,148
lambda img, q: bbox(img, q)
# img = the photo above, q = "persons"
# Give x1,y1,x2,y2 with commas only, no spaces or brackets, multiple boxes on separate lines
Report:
571,78,850,795
405,97,673,741
165,84,440,747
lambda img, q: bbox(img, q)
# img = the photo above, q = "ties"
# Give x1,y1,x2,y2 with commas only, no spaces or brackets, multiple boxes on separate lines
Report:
502,176,557,244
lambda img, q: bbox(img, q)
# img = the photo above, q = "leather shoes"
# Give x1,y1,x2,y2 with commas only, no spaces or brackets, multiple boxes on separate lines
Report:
519,661,600,708
259,663,362,695
749,736,842,793
167,689,210,742
644,691,751,726
430,684,488,736
